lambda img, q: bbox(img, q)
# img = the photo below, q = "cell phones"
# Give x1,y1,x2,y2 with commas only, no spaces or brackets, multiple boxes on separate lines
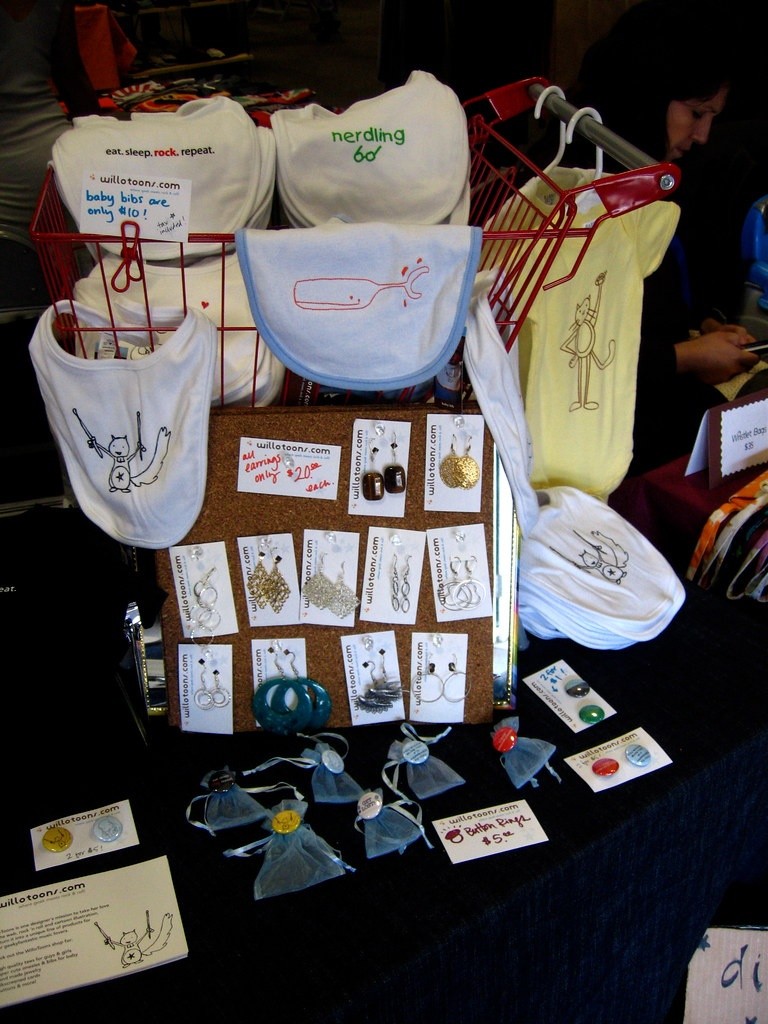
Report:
740,340,768,355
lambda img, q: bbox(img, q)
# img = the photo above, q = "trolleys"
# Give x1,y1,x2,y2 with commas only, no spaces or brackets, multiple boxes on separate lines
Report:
27,75,683,405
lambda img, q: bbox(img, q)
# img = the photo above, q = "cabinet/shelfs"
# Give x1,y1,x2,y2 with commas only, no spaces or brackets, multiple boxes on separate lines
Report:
110,0,256,82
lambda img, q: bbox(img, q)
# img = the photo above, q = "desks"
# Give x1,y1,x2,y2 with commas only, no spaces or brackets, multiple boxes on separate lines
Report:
0,500,768,1024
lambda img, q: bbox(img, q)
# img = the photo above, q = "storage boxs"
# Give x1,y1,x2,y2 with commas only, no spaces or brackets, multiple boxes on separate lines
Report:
154,406,519,734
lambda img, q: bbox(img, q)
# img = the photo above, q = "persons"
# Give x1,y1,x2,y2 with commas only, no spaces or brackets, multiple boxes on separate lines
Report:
562,2,760,543
0,0,92,308
